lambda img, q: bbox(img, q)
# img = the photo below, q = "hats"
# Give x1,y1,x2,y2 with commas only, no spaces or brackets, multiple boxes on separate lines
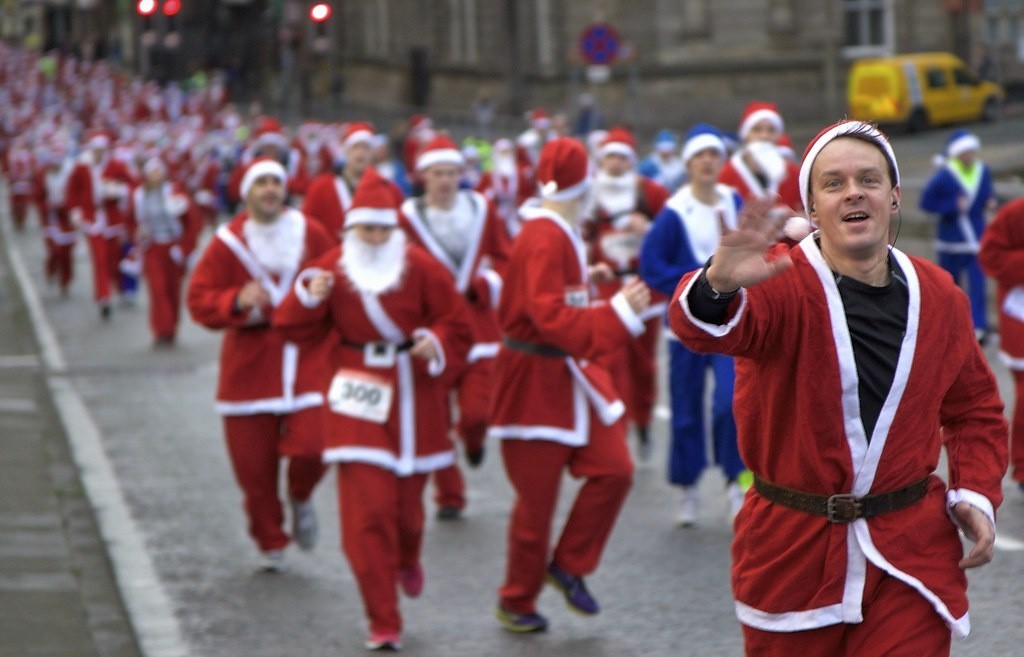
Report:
338,120,375,153
783,117,902,245
594,124,638,166
681,121,729,165
409,114,431,131
341,164,403,228
737,100,785,141
932,129,983,168
775,133,797,159
226,156,289,208
533,135,593,204
654,130,677,154
414,129,467,175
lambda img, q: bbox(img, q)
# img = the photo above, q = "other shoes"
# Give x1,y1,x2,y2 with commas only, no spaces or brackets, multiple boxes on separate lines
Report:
725,479,744,530
635,426,654,462
438,503,458,518
289,487,320,553
676,482,701,526
467,442,483,466
259,548,288,574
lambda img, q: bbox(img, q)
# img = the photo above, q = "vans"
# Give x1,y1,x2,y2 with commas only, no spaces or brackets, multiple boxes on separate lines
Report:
846,52,1006,132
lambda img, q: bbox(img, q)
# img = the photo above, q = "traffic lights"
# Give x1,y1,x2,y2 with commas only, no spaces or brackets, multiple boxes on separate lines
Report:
138,0,156,29
163,0,179,31
311,0,330,32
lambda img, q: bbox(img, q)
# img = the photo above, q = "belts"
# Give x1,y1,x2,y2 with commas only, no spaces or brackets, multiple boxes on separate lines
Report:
340,337,415,355
752,470,929,526
502,338,570,358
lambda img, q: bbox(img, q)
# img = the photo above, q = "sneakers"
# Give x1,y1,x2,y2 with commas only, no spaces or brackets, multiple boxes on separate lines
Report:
396,560,423,598
494,603,545,632
363,627,402,652
544,560,600,617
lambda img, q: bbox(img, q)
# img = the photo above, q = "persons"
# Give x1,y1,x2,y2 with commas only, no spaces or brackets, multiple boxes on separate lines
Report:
979,196,1024,491
917,128,1007,347
0,37,806,651
668,114,1010,657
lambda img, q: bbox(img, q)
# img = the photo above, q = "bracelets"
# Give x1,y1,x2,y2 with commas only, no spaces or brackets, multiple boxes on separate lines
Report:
700,254,741,299
